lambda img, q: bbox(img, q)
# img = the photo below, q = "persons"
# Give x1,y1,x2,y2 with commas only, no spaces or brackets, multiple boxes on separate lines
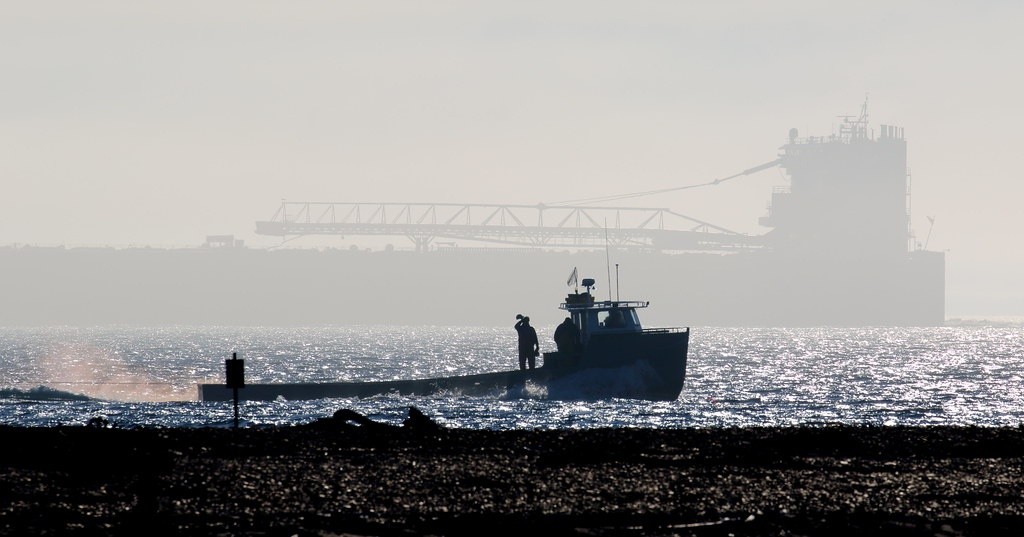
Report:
554,317,579,349
515,317,539,370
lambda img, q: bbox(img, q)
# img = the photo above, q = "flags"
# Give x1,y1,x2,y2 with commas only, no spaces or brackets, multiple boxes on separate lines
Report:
567,268,578,286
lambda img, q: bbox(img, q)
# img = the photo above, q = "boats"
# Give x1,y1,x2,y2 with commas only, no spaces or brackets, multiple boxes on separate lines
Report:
196,364,544,404
543,217,690,400
